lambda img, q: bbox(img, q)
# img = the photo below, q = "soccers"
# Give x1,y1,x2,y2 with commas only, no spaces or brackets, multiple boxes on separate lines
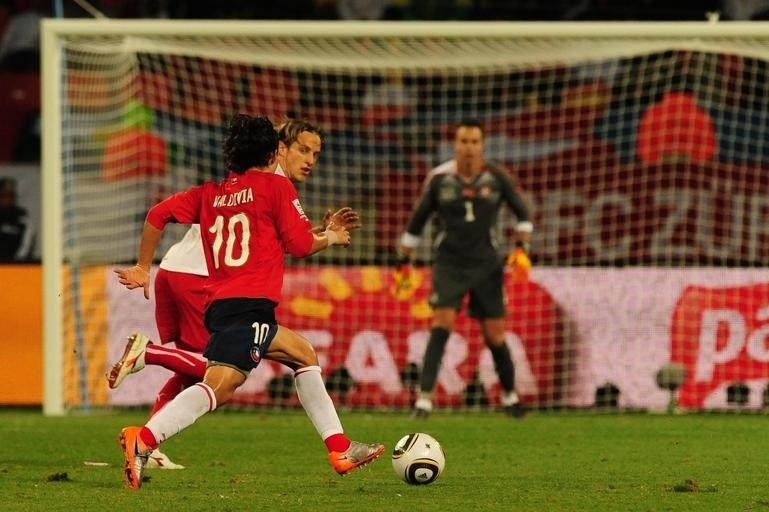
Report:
392,432,446,484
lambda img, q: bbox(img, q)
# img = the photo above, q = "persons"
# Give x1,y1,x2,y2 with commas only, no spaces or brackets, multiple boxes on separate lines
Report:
1,0,769,269
108,117,365,471
115,112,386,490
397,117,534,418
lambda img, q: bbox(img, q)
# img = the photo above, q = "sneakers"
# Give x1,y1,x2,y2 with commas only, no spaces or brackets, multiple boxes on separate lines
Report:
108,333,153,388
412,393,526,421
143,451,185,471
328,441,385,476
118,425,153,490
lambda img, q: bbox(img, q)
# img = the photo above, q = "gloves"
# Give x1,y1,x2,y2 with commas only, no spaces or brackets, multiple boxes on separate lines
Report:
391,257,413,293
508,240,532,283
